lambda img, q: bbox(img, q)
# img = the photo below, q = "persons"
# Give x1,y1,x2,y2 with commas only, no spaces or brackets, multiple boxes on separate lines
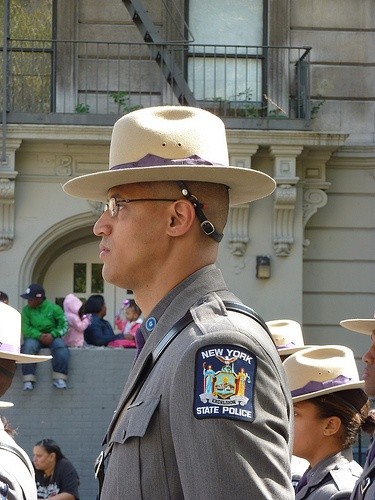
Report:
0,283,375,500
61,106,295,500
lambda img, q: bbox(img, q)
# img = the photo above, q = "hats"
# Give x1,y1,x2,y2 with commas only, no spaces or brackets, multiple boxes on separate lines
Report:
0,300,53,364
339,312,375,335
264,319,313,356
281,344,366,404
19,283,45,301
60,105,277,207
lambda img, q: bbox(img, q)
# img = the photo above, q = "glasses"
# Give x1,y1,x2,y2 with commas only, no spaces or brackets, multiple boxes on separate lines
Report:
102,196,178,217
